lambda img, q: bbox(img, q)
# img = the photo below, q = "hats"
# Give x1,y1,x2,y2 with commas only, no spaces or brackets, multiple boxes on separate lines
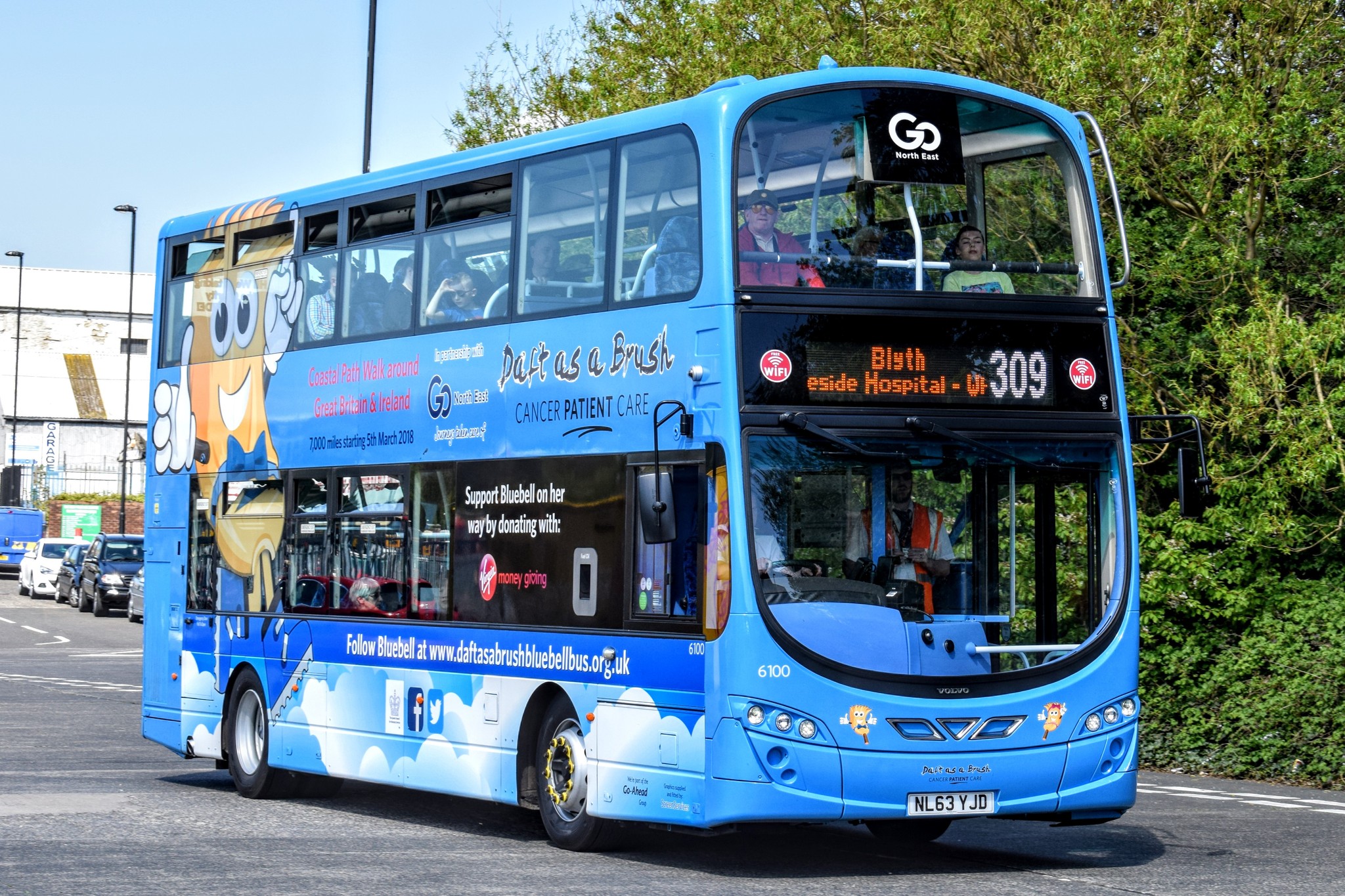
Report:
745,189,779,211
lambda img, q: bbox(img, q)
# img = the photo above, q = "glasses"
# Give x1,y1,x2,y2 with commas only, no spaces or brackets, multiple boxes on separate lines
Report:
748,204,776,214
451,289,473,296
366,592,380,599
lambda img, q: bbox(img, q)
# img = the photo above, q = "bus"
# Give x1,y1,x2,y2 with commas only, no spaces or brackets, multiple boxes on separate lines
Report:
141,49,1221,858
0,504,49,579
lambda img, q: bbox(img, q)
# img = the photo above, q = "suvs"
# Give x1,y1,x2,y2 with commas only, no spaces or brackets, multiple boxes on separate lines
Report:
75,532,145,618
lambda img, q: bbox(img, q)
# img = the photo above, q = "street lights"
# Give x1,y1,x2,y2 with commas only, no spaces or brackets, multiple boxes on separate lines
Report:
4,252,26,508
114,203,136,535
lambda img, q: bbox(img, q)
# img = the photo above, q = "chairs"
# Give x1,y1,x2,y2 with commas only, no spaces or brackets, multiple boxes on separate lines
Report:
302,215,935,346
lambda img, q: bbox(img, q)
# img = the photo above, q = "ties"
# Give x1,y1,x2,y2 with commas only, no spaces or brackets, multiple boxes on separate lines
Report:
893,509,912,550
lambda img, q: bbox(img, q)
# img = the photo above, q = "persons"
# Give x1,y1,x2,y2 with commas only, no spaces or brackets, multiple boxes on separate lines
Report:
843,471,954,615
384,235,452,330
349,577,383,610
432,570,449,620
738,189,825,287
527,235,590,298
306,263,369,340
840,226,935,289
426,272,494,323
942,225,1015,293
709,495,822,589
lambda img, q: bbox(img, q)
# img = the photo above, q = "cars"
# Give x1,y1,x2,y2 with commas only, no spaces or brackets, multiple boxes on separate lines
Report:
17,537,92,599
54,543,92,608
125,564,145,622
278,573,444,625
